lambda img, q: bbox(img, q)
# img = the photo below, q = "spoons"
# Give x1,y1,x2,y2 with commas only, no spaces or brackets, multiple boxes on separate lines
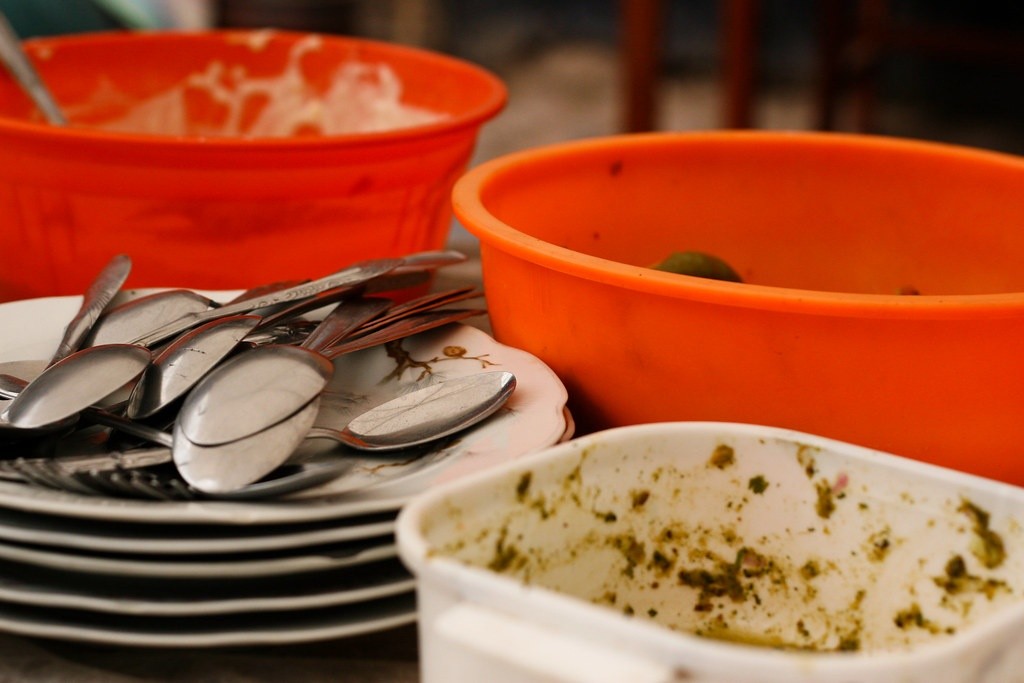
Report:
0,253,515,496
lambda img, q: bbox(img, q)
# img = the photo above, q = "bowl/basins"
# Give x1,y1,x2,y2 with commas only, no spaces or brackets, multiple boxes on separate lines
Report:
397,421,1023,683
452,130,1023,486
0,30,510,302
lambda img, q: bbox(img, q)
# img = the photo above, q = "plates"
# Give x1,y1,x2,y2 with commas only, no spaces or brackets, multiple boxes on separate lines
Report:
0,289,575,644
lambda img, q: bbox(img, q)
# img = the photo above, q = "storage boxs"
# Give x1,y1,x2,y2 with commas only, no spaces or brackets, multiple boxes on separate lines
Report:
390,422,1023,682
